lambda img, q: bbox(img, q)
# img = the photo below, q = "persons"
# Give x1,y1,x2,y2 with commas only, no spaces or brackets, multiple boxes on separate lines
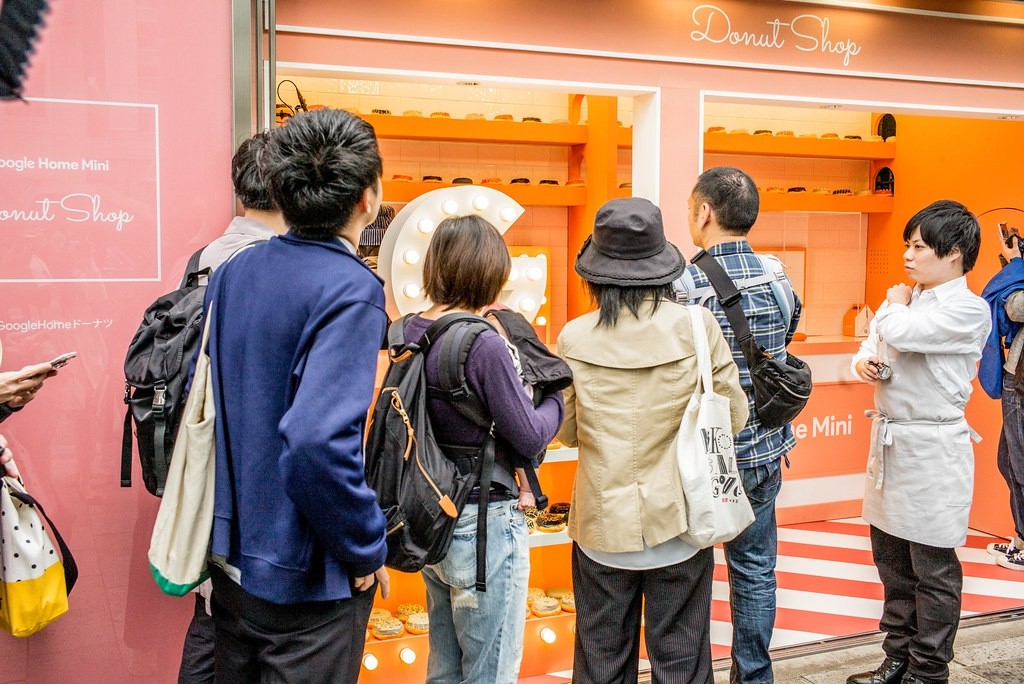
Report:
847,199,994,684
396,216,565,684
553,198,750,684
670,167,802,684
177,108,391,684
0,362,58,480
987,232,1024,571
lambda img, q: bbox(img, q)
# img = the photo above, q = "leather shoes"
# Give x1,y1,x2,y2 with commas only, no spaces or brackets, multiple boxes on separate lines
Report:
846,657,909,684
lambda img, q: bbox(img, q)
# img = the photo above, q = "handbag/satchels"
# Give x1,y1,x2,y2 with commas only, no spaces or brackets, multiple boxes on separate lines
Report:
678,306,755,548
750,349,812,429
0,448,78,637
147,296,221,598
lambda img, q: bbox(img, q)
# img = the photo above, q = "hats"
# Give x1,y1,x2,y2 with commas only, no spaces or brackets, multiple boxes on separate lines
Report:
577,198,685,287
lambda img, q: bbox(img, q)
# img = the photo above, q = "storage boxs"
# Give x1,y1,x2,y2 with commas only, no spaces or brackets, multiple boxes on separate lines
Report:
842,303,875,338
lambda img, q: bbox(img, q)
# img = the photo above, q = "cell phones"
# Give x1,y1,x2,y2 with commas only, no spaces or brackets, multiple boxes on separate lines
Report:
997,223,1010,243
21,352,78,380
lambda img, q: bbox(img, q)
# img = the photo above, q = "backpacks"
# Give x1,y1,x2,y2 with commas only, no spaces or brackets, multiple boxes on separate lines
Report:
120,240,270,498
364,312,496,572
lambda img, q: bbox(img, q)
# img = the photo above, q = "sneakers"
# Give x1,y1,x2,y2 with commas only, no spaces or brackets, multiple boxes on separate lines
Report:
987,541,1020,557
995,553,1024,571
